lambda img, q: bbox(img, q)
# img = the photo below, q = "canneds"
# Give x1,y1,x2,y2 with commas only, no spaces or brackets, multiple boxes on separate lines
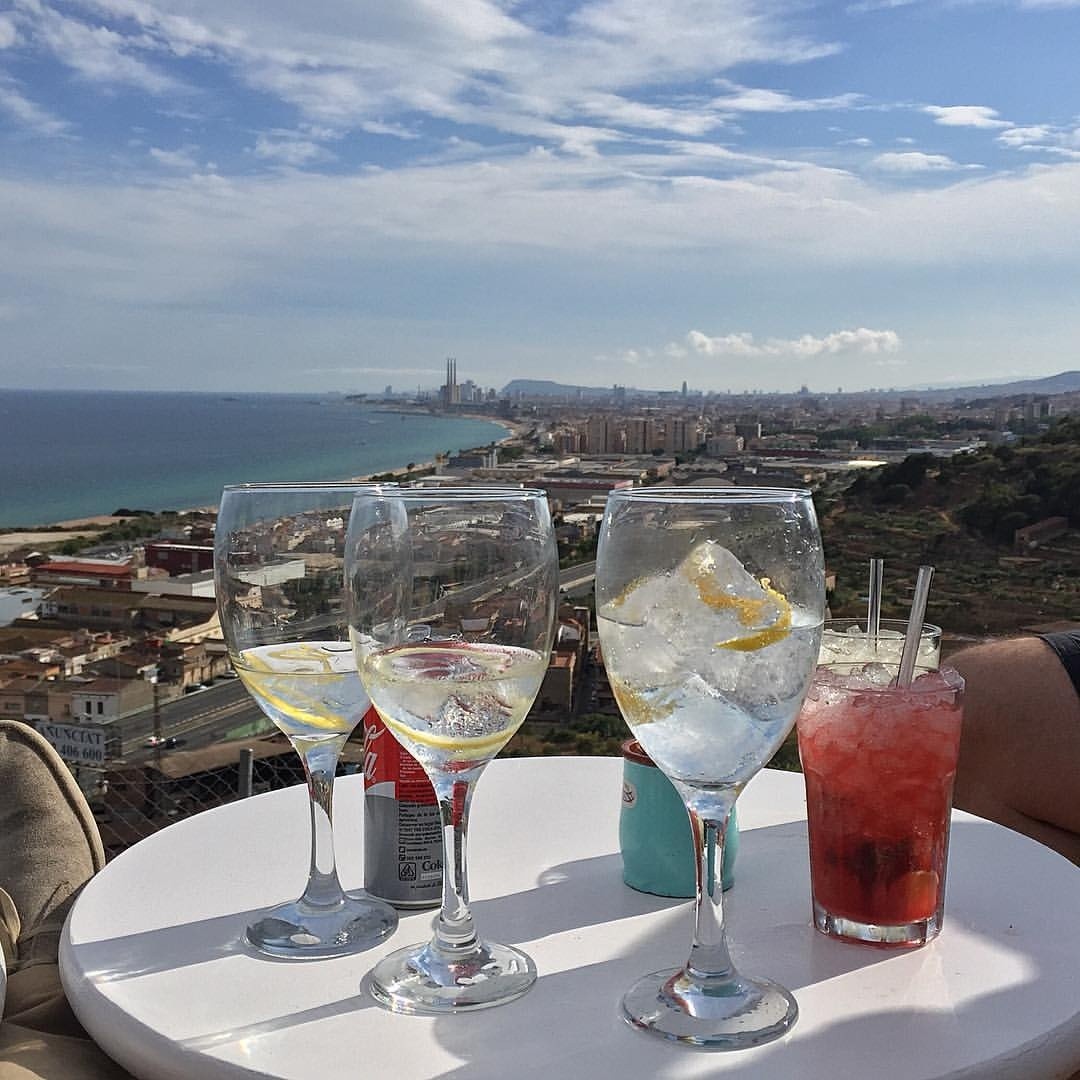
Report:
363,625,470,913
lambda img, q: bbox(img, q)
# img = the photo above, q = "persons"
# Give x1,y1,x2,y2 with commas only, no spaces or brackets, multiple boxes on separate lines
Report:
939,629,1079,867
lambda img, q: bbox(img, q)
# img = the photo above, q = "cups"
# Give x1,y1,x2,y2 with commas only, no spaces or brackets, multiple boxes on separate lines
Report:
817,616,941,679
796,662,967,950
619,737,740,900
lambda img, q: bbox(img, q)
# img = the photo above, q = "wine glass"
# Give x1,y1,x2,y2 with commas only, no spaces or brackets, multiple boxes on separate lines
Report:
595,486,827,1050
343,488,561,1016
213,481,412,965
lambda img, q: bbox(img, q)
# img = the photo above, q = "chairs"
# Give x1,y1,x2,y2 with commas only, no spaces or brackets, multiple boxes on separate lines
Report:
0,720,132,1080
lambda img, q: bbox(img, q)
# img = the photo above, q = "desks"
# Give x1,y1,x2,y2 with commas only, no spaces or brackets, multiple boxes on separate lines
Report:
59,756,1079,1080
